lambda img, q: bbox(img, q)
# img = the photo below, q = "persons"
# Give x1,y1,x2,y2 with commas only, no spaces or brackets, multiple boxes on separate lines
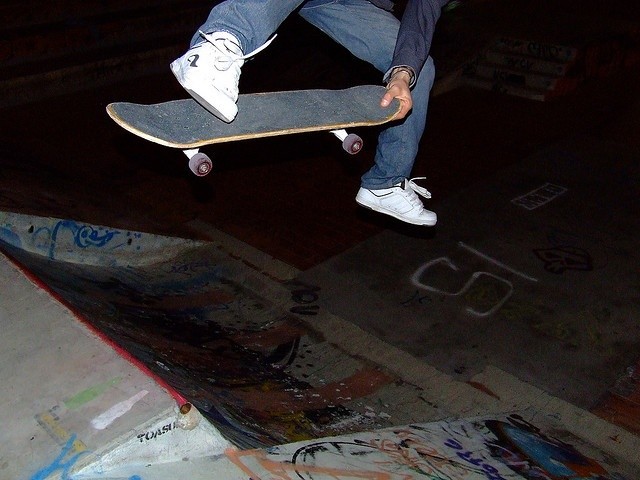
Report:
170,0,449,228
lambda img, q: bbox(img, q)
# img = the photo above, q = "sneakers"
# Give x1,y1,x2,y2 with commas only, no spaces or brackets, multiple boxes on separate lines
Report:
169,29,278,124
355,176,437,227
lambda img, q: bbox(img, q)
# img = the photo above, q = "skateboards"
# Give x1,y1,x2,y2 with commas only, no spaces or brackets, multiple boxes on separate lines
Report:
106,84,402,177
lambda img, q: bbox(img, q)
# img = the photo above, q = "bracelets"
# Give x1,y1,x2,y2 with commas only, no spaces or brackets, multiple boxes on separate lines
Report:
391,69,412,81
